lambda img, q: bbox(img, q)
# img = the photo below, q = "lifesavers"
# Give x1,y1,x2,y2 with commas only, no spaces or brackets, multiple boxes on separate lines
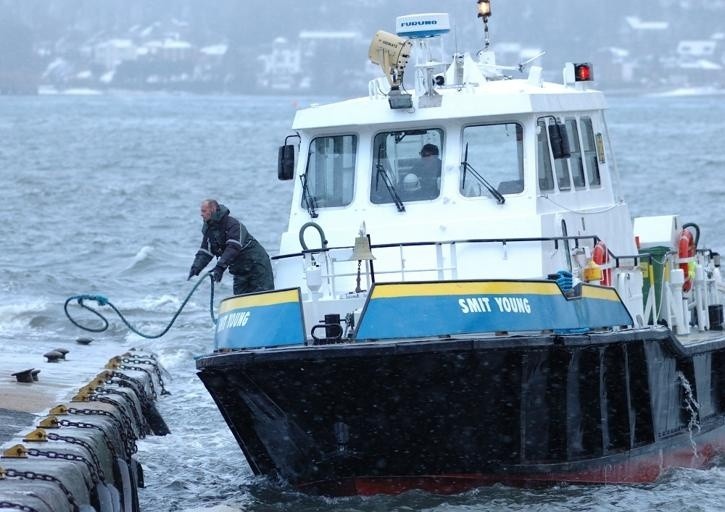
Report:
679,229,695,292
593,241,611,286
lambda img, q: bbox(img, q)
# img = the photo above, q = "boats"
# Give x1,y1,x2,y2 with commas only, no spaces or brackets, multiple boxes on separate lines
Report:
179,0,725,493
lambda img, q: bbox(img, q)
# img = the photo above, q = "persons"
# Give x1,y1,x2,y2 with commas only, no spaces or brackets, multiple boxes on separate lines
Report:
186,197,276,296
393,142,442,197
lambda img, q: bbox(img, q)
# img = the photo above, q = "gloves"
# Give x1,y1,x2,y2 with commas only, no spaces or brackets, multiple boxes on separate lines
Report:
207,265,225,283
186,267,200,281
